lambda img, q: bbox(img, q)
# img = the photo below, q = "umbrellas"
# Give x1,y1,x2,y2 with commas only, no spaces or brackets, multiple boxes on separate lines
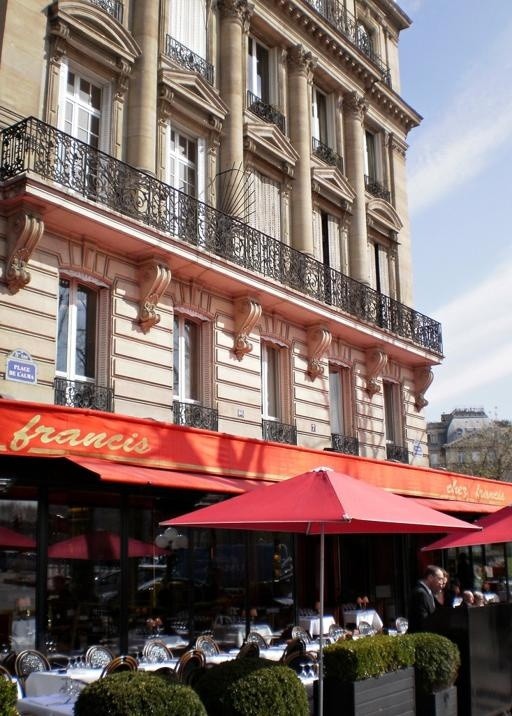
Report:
48,528,171,562
1,527,35,549
160,467,484,715
420,505,512,551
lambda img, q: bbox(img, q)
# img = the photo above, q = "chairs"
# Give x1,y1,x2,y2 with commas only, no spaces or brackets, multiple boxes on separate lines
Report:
214,605,409,700
1,635,221,716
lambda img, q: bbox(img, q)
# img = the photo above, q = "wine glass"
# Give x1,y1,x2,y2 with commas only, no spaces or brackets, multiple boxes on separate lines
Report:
134,650,168,665
59,676,79,704
299,663,319,680
66,654,94,672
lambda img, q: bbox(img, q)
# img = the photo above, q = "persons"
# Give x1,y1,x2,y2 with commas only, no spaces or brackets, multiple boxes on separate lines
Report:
409,562,500,634
